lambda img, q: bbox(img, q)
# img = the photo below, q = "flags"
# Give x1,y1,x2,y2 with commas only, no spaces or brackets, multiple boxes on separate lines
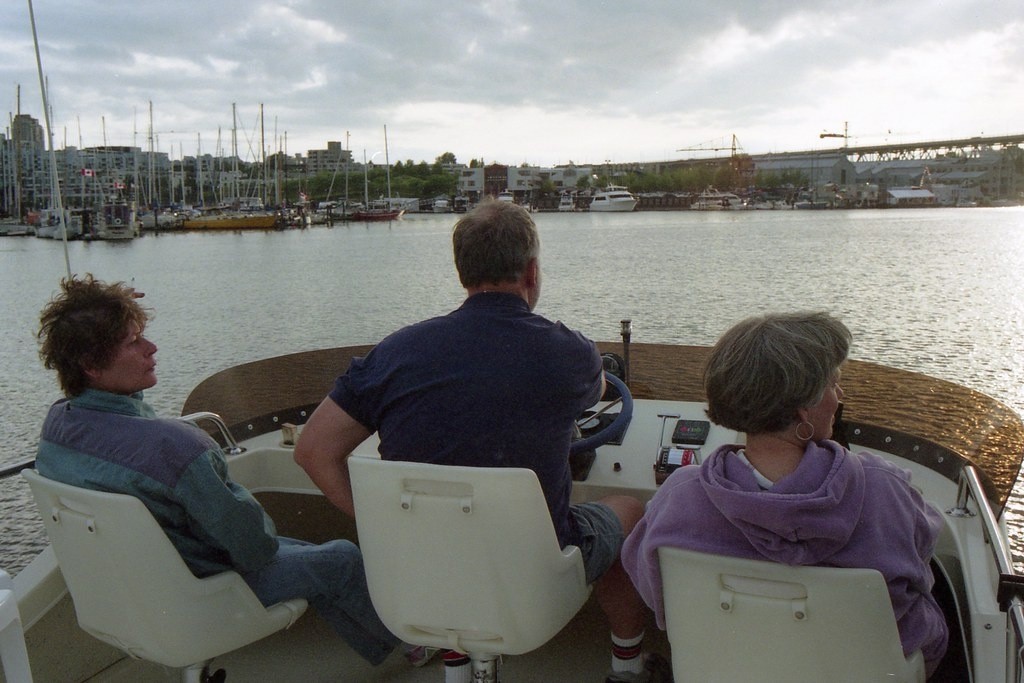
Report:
82,168,94,177
114,181,126,189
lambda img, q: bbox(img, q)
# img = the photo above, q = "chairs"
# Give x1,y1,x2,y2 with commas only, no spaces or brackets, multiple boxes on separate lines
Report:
22,469,309,683
658,546,926,683
347,455,594,683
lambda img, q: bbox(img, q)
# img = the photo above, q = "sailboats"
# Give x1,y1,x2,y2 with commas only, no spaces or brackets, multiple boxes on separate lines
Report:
0,76,399,239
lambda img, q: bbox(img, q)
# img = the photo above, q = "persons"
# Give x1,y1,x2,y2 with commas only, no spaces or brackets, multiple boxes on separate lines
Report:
33,272,440,668
293,199,655,682
621,310,950,683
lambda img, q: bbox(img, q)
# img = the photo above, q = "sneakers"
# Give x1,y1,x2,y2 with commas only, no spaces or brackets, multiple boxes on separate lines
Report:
404,646,443,668
605,652,673,683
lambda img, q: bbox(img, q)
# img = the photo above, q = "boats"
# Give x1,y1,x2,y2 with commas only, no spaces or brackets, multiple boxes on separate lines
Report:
689,184,799,210
558,185,639,212
0,342,1024,683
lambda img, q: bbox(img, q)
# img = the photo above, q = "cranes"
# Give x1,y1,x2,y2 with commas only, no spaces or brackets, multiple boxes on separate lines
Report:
676,134,743,157
820,122,848,149
